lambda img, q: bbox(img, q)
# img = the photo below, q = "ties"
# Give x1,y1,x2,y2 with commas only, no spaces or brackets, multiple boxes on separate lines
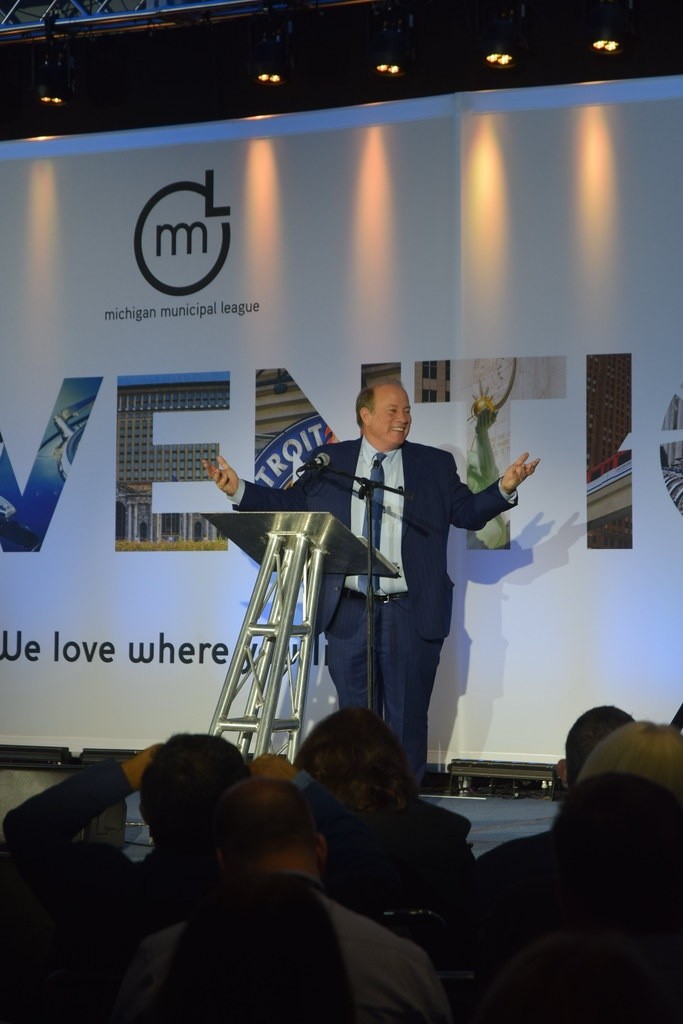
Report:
357,452,387,596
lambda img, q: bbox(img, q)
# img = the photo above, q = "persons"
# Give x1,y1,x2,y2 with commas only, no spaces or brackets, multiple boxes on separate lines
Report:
201,377,541,787
0,704,682,1024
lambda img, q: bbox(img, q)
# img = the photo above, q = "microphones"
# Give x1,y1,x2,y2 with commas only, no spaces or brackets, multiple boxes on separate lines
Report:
303,452,331,470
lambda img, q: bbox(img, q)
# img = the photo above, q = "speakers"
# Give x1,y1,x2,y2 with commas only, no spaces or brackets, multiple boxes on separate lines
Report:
0,757,130,852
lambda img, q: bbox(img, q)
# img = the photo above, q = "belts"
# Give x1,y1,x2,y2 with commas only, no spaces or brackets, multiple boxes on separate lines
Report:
341,587,408,601
